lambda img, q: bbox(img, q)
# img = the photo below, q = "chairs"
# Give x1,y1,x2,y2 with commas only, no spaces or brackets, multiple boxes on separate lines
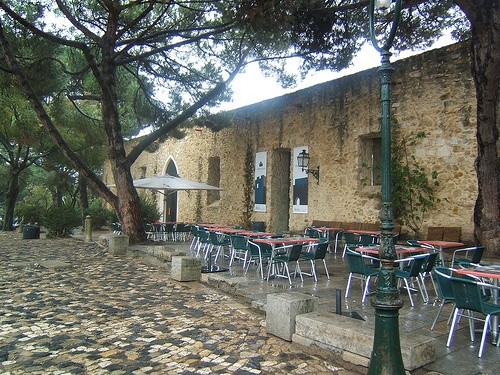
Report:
112,222,500,357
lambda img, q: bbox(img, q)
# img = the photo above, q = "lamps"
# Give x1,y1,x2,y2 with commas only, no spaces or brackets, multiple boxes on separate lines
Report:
297,149,319,185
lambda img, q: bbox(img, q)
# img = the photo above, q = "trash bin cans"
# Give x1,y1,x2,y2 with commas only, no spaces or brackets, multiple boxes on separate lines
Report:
23,225,40,239
252,221,265,232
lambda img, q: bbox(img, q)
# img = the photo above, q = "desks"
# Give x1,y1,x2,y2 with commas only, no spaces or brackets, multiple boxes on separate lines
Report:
198,223,234,228
315,228,344,254
456,267,500,346
253,237,320,280
355,246,427,293
346,230,380,242
208,228,246,234
238,232,277,268
418,240,464,267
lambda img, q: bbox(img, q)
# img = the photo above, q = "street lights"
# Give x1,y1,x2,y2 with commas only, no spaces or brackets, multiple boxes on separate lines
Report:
360,0,407,375
